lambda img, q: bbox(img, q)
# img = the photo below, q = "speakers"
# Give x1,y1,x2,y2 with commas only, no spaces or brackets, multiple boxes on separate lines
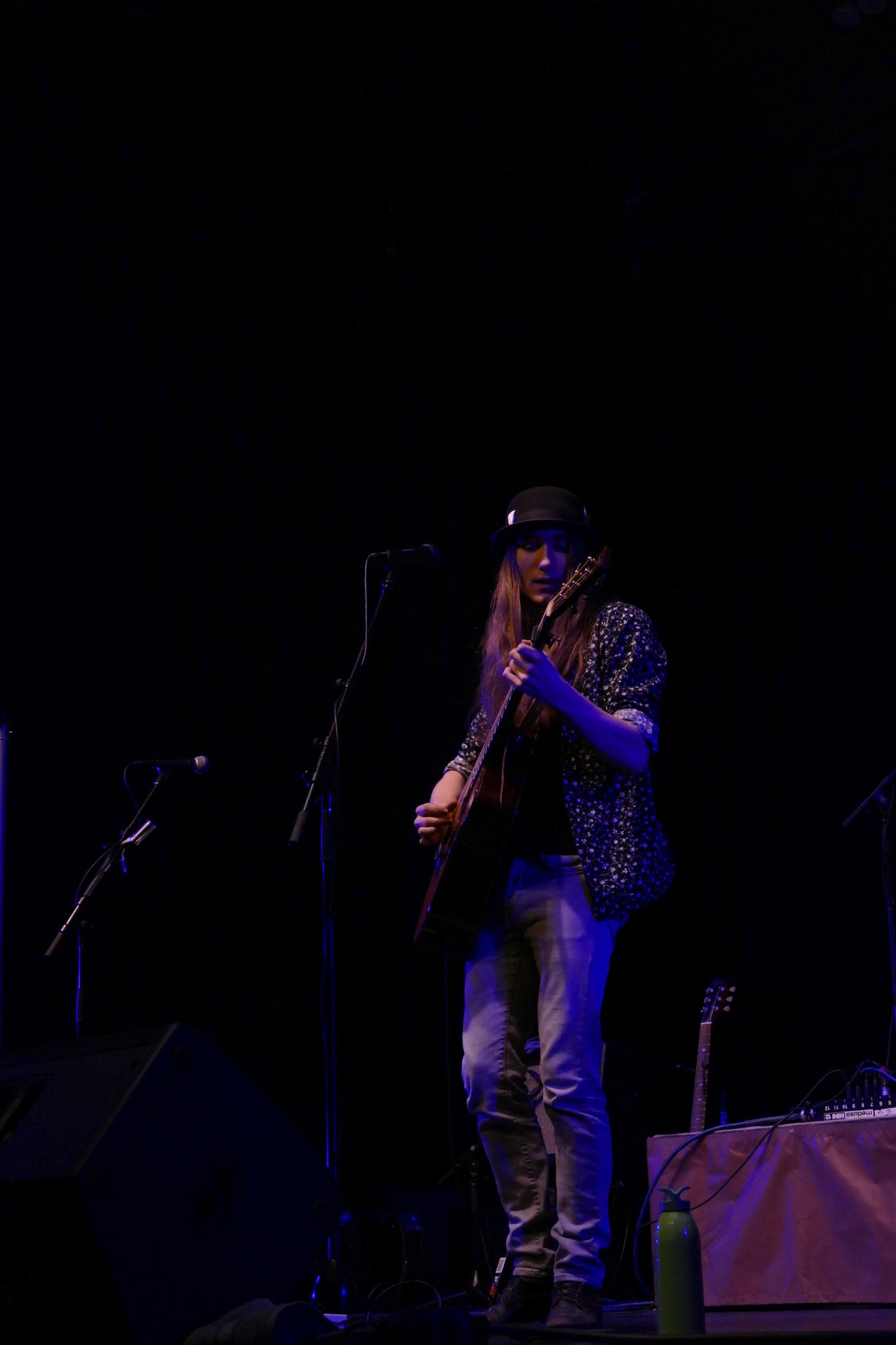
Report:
0,1022,357,1345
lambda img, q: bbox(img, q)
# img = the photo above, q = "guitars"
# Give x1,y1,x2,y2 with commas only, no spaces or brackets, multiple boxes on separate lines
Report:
688,972,739,1135
410,541,621,953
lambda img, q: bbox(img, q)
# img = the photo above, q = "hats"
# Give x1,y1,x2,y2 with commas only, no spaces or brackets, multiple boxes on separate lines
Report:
490,486,591,557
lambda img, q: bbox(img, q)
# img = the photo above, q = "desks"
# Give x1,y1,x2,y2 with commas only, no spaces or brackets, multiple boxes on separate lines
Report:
645,1118,896,1307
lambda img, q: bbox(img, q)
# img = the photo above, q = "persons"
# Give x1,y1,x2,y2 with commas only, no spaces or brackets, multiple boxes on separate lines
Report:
413,485,667,1323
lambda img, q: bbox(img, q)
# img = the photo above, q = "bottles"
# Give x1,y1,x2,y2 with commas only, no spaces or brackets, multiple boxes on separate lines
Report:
655,1185,706,1335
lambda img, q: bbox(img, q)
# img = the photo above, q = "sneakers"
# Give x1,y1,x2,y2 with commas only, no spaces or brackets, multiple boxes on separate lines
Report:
484,1275,553,1324
545,1281,603,1329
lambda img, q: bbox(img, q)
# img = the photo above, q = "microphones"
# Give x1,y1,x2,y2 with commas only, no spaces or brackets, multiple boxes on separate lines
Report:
133,756,211,776
369,543,439,561
